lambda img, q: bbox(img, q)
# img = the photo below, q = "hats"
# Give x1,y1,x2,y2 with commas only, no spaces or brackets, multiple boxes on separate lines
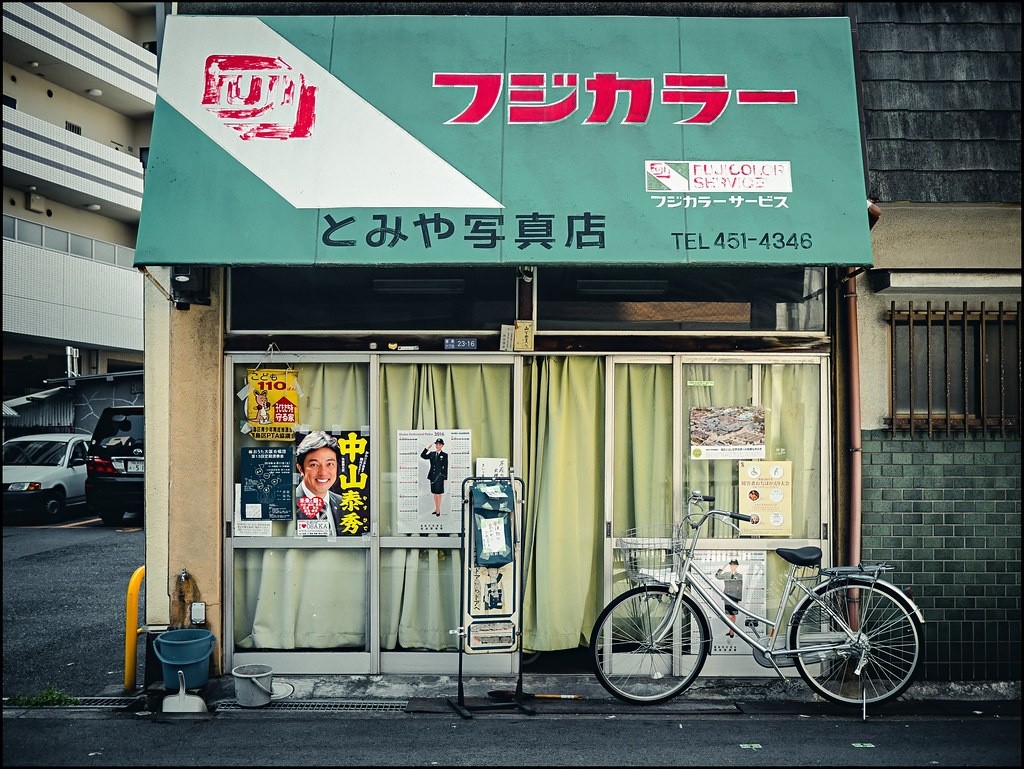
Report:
434,438,444,445
729,559,739,565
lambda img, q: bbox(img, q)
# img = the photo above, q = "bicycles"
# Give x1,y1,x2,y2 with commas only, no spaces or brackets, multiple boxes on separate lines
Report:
589,490,926,722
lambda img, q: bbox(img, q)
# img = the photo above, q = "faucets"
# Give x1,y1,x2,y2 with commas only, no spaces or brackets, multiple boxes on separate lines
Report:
180,568,189,582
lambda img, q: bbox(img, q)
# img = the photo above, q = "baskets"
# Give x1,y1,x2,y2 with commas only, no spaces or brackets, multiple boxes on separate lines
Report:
620,523,688,585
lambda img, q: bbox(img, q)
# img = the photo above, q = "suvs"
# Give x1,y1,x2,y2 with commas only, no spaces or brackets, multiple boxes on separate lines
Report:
85,405,145,528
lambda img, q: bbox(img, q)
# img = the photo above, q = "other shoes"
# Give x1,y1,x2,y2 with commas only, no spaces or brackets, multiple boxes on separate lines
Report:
730,633,734,638
436,513,440,517
432,511,436,514
726,633,730,636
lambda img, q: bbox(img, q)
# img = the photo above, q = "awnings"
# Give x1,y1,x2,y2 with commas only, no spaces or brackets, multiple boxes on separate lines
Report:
134,15,874,268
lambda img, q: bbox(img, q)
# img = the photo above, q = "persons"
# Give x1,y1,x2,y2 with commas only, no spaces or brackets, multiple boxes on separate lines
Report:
715,559,743,638
296,431,351,536
421,438,448,517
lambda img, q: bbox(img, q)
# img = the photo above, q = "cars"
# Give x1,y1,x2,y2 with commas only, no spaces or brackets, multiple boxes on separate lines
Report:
2,432,92,524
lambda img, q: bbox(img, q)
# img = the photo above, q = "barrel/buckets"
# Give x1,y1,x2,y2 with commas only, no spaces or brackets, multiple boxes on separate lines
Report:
153,629,216,689
232,665,275,706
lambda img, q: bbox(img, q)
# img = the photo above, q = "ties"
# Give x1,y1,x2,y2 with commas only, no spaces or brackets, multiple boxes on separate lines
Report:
437,452,438,456
318,501,328,520
731,574,734,579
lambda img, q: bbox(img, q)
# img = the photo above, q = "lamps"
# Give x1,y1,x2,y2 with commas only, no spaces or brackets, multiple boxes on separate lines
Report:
88,89,103,97
87,204,101,211
